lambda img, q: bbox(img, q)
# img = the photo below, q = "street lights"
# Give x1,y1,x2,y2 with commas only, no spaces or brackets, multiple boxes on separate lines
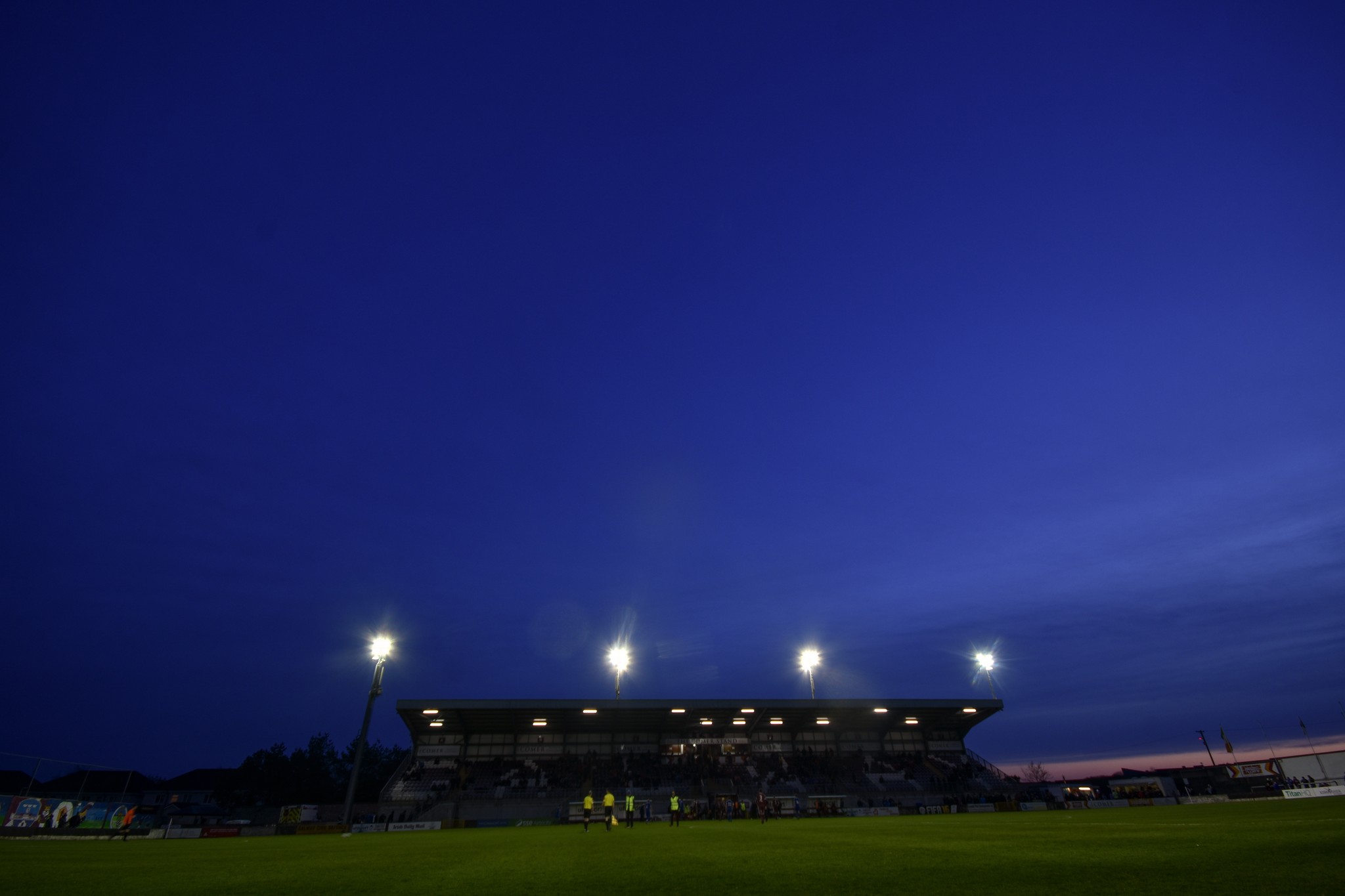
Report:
976,654,996,700
335,636,392,831
610,648,630,698
800,650,816,699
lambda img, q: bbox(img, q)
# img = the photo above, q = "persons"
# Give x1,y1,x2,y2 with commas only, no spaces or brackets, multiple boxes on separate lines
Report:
337,811,407,831
639,799,653,824
668,791,680,826
114,804,139,842
680,789,782,825
625,789,634,828
402,740,1046,815
792,793,922,820
582,790,594,832
44,811,81,834
601,788,614,830
1044,775,1333,801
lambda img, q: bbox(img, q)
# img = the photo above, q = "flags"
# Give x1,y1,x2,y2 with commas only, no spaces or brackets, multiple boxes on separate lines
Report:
1299,720,1306,735
1219,728,1233,753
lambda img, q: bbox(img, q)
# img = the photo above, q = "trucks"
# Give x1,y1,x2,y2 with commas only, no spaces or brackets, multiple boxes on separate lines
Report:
279,805,321,824
